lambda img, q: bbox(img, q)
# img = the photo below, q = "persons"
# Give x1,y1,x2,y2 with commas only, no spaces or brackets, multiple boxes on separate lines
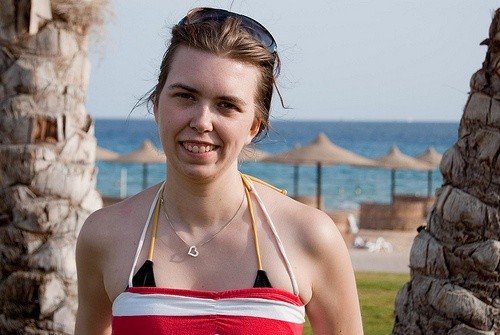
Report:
74,6,365,335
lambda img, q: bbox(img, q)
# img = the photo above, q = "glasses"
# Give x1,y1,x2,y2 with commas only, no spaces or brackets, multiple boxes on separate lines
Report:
176,8,277,55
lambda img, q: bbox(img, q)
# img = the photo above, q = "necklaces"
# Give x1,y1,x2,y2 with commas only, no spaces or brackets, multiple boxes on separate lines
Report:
160,188,246,258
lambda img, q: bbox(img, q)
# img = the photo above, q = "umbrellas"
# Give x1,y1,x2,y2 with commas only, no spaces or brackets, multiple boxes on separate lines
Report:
97,138,166,190
238,131,443,211
